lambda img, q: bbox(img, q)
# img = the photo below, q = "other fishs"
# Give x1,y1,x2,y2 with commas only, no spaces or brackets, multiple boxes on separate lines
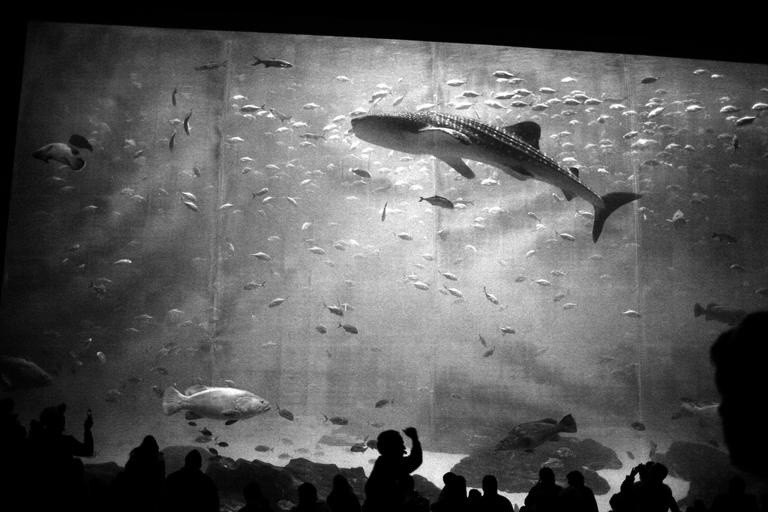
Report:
1,44,765,470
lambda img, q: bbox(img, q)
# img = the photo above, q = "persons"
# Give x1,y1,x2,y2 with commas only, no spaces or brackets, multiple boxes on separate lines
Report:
710,309,768,512
